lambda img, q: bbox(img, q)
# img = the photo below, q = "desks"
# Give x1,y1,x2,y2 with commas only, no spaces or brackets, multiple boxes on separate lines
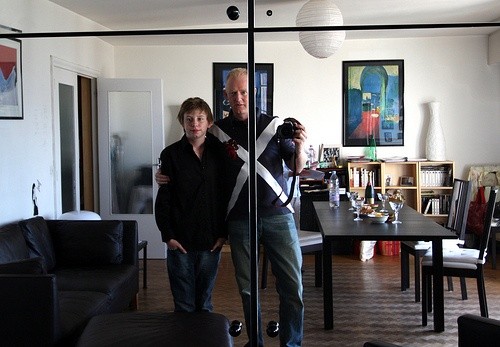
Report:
313,201,459,332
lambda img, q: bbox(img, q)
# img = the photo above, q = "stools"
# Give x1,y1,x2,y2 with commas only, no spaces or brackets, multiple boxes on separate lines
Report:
78,312,234,347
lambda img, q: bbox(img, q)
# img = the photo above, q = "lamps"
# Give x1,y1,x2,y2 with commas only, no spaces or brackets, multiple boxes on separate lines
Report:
296,0,345,58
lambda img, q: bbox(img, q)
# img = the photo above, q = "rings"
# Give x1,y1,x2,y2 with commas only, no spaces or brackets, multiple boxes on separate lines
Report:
158,182,160,184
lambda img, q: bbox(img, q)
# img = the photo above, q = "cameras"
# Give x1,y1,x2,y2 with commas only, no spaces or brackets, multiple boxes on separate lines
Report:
276,118,302,139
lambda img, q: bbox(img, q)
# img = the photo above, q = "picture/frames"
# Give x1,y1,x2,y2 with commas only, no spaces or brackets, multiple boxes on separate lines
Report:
321,146,341,162
212,62,274,121
0,37,23,119
342,59,404,147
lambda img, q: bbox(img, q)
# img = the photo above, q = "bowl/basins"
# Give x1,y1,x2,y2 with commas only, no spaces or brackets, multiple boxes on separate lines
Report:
367,214,389,223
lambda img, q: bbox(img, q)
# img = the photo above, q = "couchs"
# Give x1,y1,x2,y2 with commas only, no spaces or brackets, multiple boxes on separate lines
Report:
0,217,139,347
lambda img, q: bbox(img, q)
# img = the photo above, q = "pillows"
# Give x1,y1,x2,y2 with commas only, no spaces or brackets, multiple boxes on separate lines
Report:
58,221,123,266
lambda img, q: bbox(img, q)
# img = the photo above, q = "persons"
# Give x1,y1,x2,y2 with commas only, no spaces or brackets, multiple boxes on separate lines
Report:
156,67,307,347
154,97,237,313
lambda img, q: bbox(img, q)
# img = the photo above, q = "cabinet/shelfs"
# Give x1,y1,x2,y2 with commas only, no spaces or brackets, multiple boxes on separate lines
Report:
348,160,455,223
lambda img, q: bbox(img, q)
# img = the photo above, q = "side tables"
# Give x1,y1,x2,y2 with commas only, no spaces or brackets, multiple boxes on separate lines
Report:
138,241,147,289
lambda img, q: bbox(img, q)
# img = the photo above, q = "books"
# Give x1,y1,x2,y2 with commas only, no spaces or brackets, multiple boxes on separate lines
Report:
422,195,451,215
350,166,380,187
421,165,453,187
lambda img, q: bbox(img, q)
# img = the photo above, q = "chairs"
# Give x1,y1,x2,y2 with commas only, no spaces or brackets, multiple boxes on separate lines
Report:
401,165,500,326
261,231,323,287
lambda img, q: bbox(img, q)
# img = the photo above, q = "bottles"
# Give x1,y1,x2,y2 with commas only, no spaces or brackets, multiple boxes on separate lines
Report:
309,145,314,163
364,171,374,204
367,135,377,161
328,171,340,207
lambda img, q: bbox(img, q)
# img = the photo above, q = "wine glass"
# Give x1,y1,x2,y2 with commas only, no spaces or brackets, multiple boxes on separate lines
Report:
346,191,358,210
352,197,364,221
388,201,404,224
377,193,390,212
